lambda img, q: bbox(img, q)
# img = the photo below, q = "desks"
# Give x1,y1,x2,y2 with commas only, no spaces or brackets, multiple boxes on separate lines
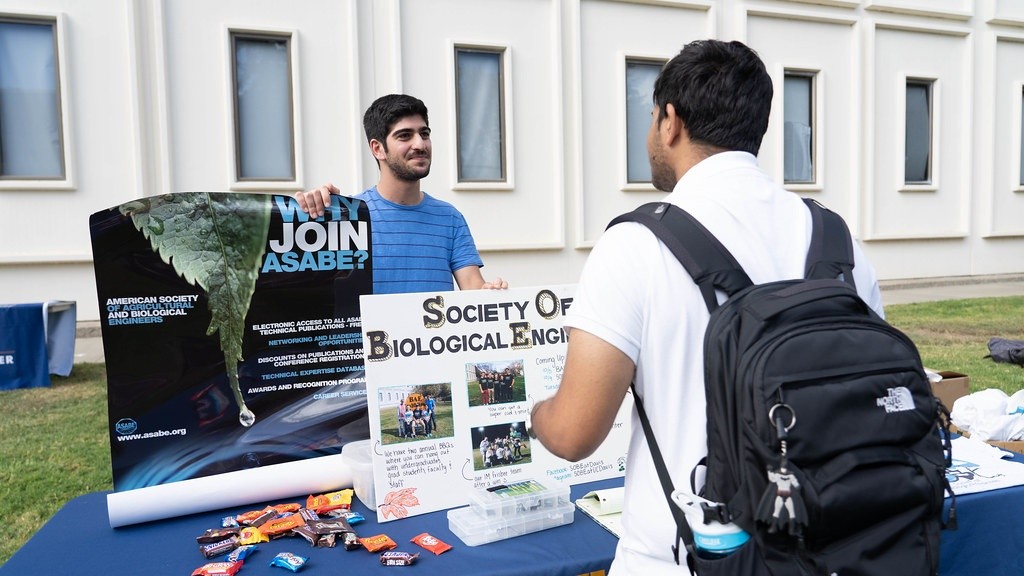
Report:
0,299,77,390
0,428,1024,576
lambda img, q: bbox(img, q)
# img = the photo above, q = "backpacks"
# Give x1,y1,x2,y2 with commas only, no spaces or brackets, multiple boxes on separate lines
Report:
601,196,958,576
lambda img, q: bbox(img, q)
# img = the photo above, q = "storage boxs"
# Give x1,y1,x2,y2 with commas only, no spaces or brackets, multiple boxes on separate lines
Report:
447,476,575,547
929,371,970,426
948,425,1024,455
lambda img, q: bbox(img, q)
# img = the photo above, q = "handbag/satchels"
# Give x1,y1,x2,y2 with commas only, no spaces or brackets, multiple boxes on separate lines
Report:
983,336,1024,367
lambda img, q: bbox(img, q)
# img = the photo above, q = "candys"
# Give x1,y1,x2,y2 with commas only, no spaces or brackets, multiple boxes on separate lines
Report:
189,488,453,576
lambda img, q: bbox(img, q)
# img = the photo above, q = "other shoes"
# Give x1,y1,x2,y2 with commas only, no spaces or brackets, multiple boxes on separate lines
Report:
427,433,432,438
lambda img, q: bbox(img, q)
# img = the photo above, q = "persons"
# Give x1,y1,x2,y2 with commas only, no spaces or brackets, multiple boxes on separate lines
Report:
479,426,526,467
397,394,437,439
530,39,886,576
479,367,516,405
294,94,508,295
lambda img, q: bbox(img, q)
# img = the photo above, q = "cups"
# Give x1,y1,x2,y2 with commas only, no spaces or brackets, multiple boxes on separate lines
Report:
685,502,749,557
